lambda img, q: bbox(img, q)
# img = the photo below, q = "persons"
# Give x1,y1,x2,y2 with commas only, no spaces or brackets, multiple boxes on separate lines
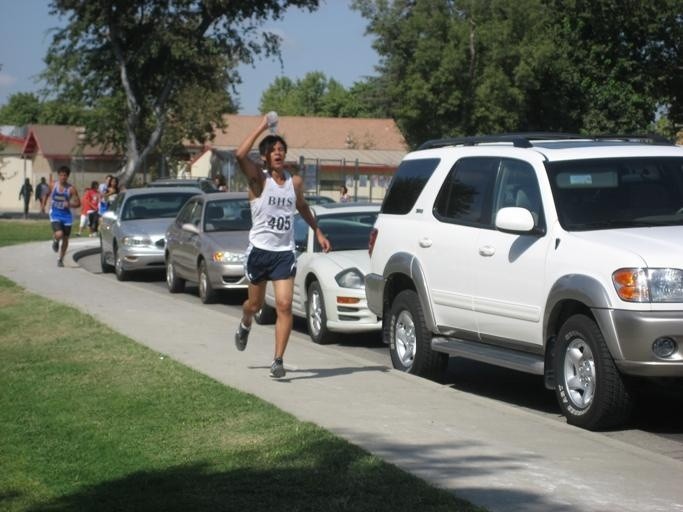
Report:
340,185,352,202
215,175,229,191
19,165,127,268
235,115,330,382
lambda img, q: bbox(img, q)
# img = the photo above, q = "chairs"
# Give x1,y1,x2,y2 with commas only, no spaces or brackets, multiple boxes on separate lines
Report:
516,187,539,226
129,206,149,219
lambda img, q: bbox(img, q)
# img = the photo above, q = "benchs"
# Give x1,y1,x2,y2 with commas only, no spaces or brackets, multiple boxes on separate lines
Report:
205,207,251,231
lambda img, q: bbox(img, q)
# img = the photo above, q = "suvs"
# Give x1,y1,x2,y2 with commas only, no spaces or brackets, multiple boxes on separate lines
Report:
365,124,683,425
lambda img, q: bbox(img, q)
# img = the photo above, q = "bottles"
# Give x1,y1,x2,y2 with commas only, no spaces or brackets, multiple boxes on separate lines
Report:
266,111,278,136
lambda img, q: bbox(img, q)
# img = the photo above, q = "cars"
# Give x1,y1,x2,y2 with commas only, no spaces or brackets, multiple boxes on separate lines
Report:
159,192,256,303
249,196,388,347
302,196,334,205
95,186,205,276
146,177,220,197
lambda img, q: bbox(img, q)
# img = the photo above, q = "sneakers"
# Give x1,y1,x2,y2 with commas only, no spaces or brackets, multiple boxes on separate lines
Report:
269,357,285,378
52,234,58,253
234,322,249,352
56,257,64,267
